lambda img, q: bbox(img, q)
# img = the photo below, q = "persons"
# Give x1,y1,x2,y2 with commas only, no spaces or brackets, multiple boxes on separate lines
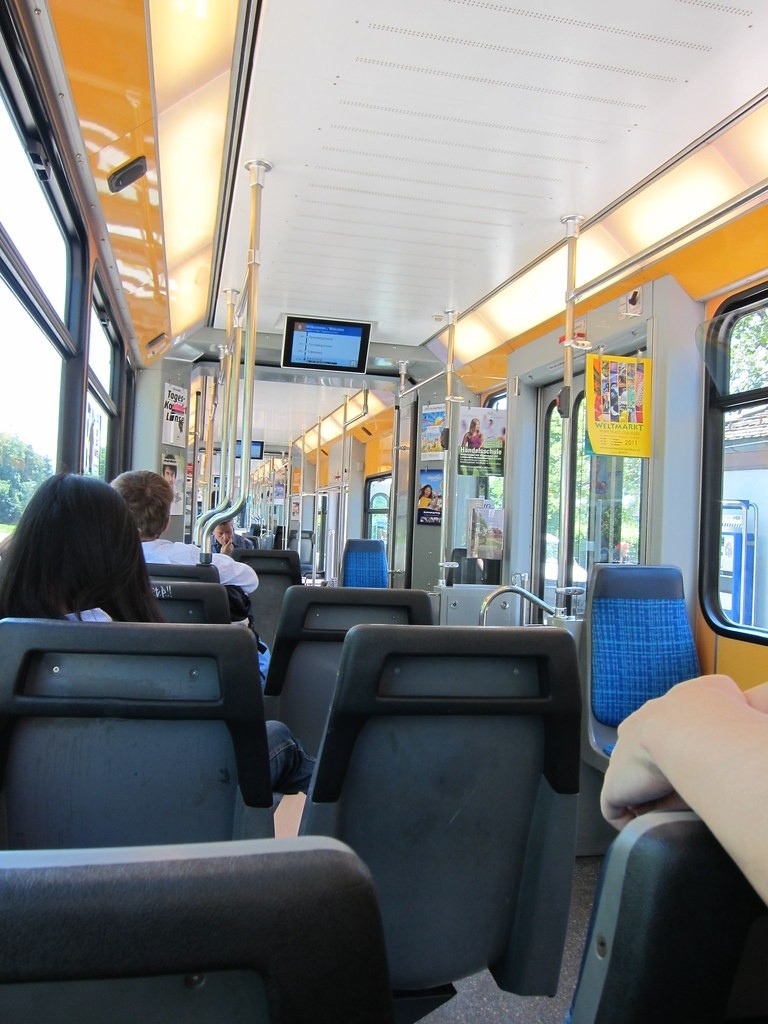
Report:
599,673,768,908
163,455,180,495
0,472,318,798
292,502,299,516
110,470,269,689
210,515,254,553
461,418,506,475
210,490,233,545
419,483,442,511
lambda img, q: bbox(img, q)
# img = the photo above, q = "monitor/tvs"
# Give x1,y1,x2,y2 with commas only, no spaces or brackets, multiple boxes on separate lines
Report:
281,314,372,374
236,440,264,460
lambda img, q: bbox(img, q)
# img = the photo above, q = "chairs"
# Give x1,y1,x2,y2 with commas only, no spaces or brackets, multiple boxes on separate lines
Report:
0,523,768,1024
